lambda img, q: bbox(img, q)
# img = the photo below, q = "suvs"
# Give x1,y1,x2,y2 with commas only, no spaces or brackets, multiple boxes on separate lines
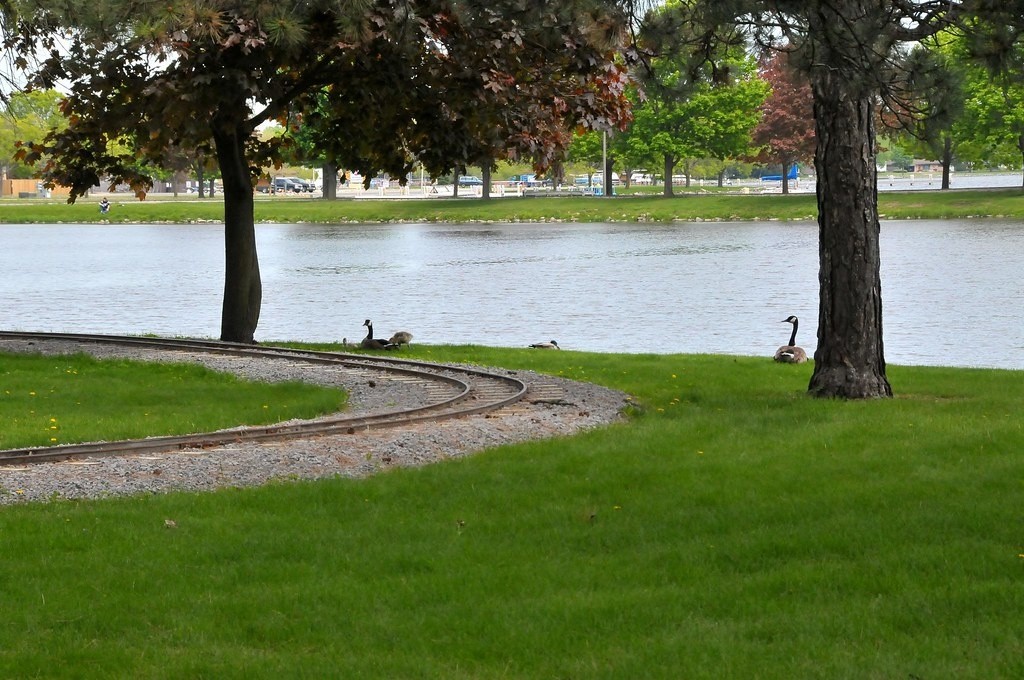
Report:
273,176,315,193
458,175,483,189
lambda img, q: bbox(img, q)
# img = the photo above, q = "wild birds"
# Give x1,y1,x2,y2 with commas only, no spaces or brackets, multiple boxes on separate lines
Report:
361,319,413,348
529,340,560,350
774,316,808,363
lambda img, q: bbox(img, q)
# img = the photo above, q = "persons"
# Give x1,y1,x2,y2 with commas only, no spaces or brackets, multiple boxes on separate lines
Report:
99,198,110,213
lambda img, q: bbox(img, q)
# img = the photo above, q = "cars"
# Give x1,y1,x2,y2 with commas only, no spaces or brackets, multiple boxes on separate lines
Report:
256,183,279,193
